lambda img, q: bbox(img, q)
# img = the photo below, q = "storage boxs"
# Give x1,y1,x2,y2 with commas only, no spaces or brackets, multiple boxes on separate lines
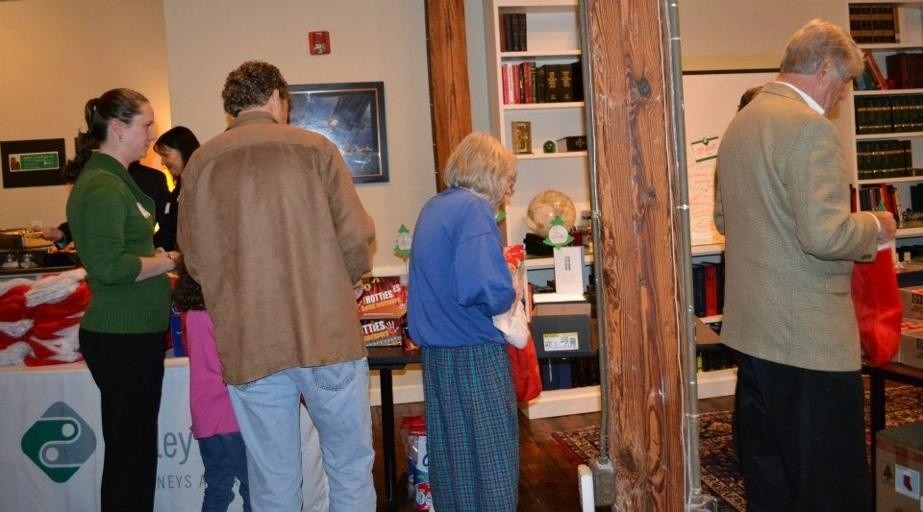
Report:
530,290,598,392
889,284,923,370
360,319,403,359
355,276,407,316
876,419,923,512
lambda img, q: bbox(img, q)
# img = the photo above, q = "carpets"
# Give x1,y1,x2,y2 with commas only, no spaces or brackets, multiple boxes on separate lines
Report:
551,385,922,512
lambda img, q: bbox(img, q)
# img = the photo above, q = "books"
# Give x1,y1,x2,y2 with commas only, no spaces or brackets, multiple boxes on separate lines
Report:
499,12,587,151
849,3,923,229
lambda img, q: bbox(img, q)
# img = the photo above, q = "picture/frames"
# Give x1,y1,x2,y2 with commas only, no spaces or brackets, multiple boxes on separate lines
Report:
286,81,389,184
0,138,67,188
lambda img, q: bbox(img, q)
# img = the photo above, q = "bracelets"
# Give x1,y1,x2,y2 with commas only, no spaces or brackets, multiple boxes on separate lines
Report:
166,253,176,264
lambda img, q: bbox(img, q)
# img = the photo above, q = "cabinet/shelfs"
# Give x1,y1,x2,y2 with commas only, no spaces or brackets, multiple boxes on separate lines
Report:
483,0,738,419
845,0,923,239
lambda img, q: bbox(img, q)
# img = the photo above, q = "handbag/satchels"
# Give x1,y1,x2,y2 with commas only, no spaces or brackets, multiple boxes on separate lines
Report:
850,248,904,367
492,300,542,402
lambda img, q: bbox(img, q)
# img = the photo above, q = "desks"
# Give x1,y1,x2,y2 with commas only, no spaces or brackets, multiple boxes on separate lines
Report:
0,252,78,279
369,346,423,511
862,358,923,474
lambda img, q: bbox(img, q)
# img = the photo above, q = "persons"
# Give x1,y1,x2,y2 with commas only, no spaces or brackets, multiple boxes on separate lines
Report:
713,19,899,511
60,87,184,511
724,81,763,476
407,132,523,512
33,161,169,252
168,252,249,512
174,60,377,512
152,125,200,199
